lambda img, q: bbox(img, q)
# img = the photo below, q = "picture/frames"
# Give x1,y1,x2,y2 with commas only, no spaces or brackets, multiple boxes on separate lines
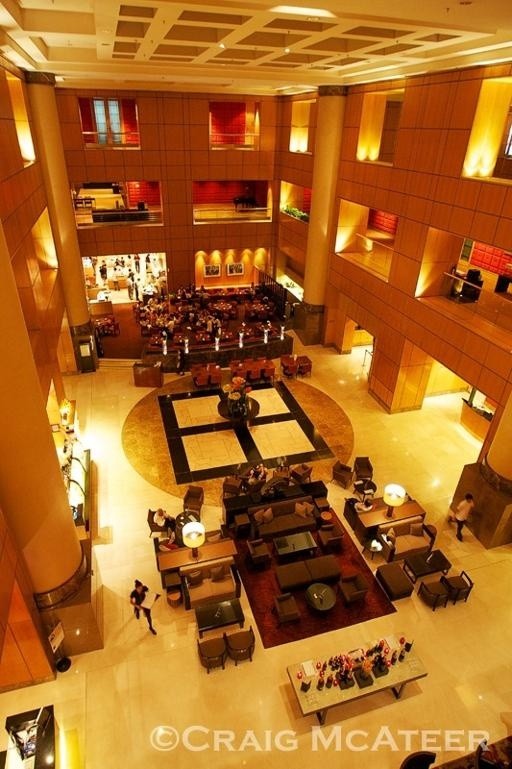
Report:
203,263,244,278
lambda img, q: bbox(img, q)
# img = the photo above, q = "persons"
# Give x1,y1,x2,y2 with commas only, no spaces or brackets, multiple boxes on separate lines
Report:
89,253,221,374
153,509,174,536
344,497,374,512
130,579,156,636
448,493,475,542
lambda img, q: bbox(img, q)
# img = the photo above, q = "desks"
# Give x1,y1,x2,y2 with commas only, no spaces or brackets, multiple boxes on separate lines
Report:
287,633,428,726
74,198,96,208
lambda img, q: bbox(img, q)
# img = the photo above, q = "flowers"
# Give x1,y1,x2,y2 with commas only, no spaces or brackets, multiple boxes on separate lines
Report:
221,377,252,406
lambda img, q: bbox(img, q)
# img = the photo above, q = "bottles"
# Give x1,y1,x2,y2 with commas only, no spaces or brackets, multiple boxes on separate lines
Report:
295,640,405,692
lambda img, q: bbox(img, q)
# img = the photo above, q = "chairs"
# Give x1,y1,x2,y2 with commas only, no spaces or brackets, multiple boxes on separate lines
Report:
147,457,474,674
76,196,92,208
175,298,276,323
96,323,120,336
